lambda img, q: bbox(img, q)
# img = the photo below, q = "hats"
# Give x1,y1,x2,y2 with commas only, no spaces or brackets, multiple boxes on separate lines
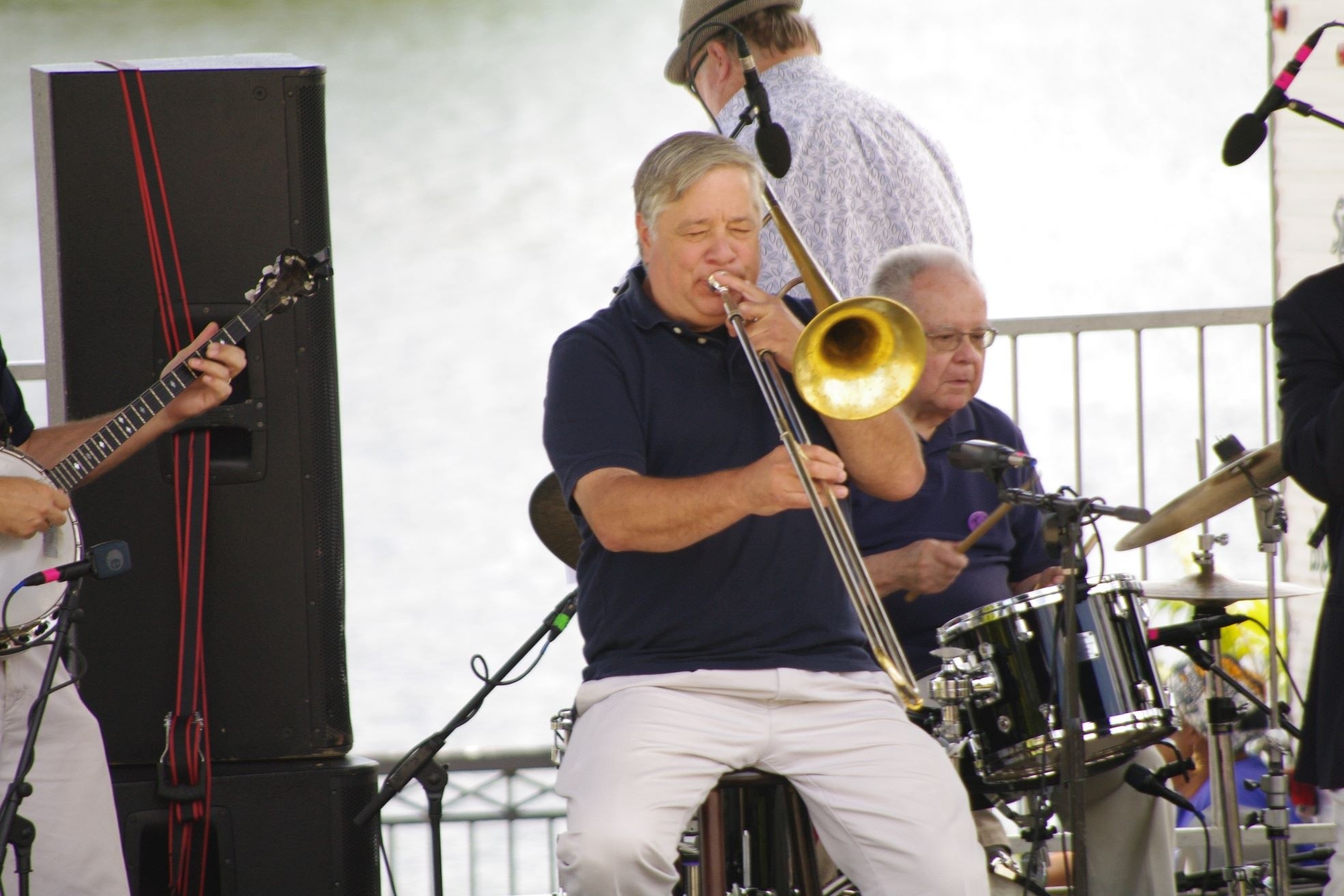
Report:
664,0,804,86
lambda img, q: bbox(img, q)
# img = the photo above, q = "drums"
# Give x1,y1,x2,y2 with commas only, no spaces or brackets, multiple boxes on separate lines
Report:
929,566,1191,798
822,856,1047,896
677,765,861,896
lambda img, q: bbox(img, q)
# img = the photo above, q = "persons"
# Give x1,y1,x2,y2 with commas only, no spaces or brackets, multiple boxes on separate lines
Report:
1271,261,1343,896
1029,657,1320,892
852,244,1181,896
0,320,247,896
659,0,975,326
543,130,992,896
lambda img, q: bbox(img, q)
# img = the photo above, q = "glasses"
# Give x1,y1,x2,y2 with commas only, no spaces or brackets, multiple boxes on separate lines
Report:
924,326,998,352
684,52,708,96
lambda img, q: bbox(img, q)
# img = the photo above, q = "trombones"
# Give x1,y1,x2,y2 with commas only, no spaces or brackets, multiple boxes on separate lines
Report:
710,175,932,716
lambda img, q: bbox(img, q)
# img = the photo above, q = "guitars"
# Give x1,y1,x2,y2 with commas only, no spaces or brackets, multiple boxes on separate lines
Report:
0,237,330,654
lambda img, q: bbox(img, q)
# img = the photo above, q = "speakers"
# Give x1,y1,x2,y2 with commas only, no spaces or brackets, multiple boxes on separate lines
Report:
29,53,358,766
107,754,380,895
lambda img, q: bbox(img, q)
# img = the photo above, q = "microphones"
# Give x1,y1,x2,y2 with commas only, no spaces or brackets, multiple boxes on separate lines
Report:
946,438,1039,474
1124,762,1192,812
24,537,130,587
1222,27,1322,166
735,33,792,178
1146,615,1248,646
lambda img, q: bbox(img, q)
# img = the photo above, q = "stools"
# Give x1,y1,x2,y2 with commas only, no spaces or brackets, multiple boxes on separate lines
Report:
689,767,823,896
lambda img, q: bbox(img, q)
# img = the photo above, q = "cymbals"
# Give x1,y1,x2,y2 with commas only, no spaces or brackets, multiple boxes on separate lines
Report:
526,469,583,572
1135,569,1329,607
1115,435,1287,555
